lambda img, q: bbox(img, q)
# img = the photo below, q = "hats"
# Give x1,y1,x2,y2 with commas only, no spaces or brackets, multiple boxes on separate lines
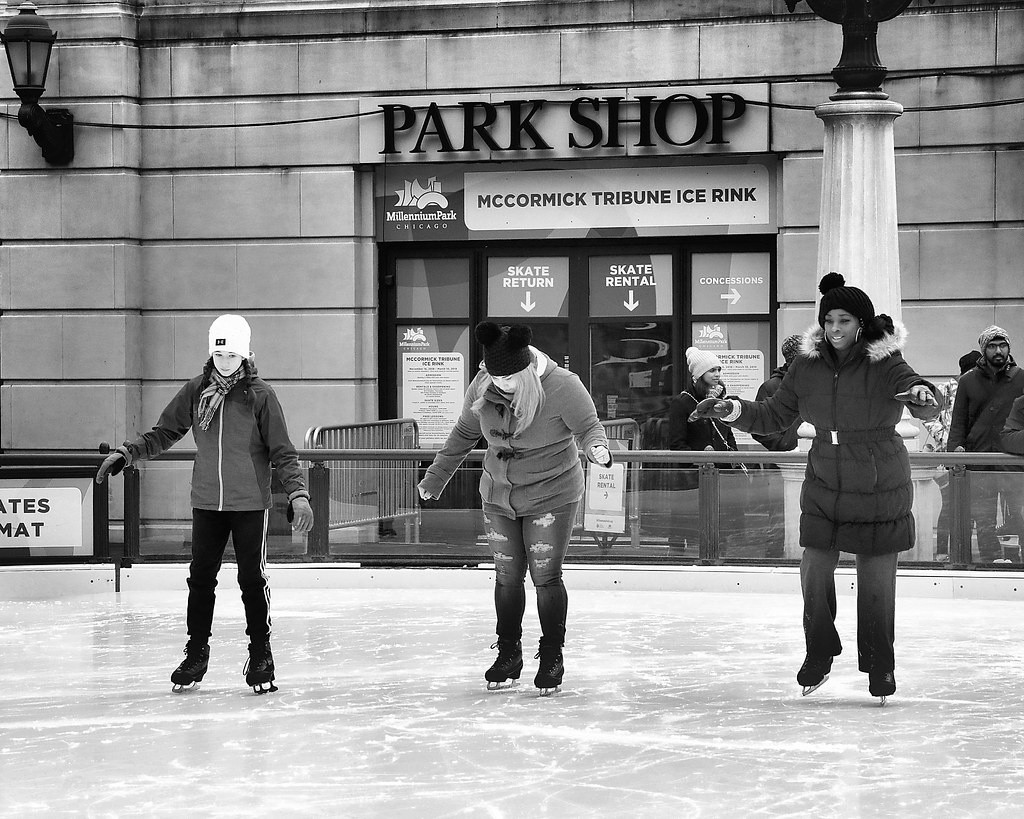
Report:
475,320,533,377
978,325,1011,364
817,271,875,328
209,314,251,360
959,351,983,376
782,335,806,366
685,346,722,380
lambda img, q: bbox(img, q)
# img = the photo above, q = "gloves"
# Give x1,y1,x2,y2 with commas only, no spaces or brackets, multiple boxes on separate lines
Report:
688,397,734,422
95,446,132,485
287,491,314,534
894,385,939,408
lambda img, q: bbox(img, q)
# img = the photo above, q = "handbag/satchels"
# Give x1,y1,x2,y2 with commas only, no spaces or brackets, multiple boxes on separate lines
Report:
728,446,749,484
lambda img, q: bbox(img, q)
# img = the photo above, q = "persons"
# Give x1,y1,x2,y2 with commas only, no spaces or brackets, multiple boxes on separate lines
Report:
669,346,738,555
96,314,314,694
417,323,613,697
922,325,1024,564
686,273,947,707
750,333,804,558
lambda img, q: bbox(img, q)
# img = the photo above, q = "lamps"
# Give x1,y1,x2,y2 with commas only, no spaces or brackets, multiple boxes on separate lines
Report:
0,0,74,166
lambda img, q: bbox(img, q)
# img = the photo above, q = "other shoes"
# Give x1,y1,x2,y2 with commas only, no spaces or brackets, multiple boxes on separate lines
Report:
997,519,1018,536
667,537,688,557
938,553,949,563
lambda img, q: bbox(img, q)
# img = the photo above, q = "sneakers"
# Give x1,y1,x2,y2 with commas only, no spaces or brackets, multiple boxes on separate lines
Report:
171,638,209,692
797,650,833,696
534,635,564,697
485,637,523,691
246,638,277,694
868,669,896,707
